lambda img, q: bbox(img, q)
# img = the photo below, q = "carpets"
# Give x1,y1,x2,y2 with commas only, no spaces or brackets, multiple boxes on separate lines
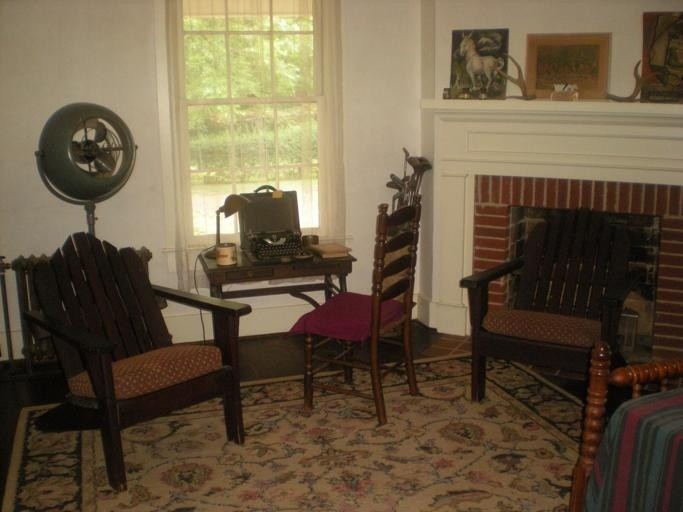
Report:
1,351,612,512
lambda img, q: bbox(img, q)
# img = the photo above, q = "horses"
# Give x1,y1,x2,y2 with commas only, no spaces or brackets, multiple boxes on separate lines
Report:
459,30,505,93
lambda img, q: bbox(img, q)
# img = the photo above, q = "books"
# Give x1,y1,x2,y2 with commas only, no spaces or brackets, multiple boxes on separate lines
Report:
309,243,353,260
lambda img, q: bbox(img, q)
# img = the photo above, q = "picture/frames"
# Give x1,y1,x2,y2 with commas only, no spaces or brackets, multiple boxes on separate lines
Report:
525,32,609,100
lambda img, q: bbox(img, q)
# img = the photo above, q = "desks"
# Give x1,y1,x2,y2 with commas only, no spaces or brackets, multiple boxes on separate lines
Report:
198,242,356,386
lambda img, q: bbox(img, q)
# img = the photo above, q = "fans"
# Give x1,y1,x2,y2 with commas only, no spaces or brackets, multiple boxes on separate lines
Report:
36,102,137,234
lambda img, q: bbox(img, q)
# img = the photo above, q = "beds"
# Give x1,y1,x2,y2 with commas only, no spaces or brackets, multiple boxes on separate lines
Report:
569,339,683,512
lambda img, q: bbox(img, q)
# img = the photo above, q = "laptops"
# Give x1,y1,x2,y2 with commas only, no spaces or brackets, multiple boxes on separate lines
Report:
239,191,314,265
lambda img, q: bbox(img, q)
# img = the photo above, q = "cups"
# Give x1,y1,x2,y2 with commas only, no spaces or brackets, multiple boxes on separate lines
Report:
215,242,237,266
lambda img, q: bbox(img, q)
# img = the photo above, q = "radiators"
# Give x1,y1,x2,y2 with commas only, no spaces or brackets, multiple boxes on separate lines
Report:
0,247,151,375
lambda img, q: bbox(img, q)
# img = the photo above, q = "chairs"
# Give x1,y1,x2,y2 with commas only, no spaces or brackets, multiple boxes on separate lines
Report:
287,191,421,423
459,207,634,402
21,233,255,491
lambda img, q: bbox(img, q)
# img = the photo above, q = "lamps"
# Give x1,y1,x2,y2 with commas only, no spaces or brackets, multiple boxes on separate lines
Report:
204,194,252,258
